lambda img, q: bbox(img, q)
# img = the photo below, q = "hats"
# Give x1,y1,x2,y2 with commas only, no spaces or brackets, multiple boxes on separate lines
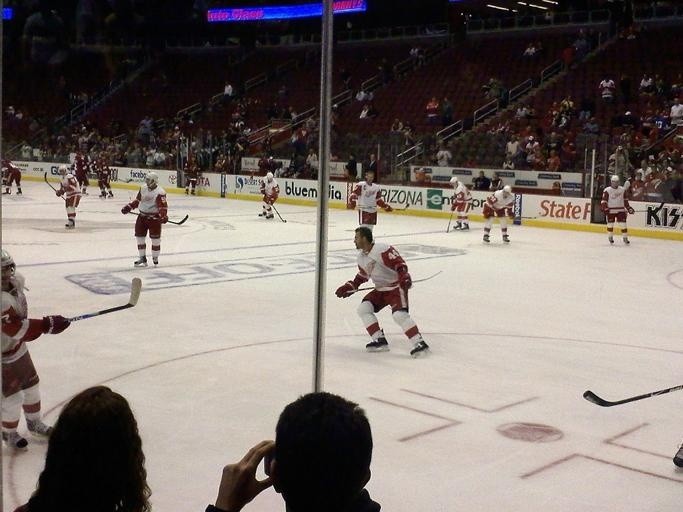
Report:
529,136,534,140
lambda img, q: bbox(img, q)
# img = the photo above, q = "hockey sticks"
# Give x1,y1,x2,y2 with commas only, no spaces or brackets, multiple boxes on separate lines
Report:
446,198,456,233
354,202,410,211
345,269,443,293
490,213,543,222
582,386,680,409
44,172,68,204
128,209,189,226
608,203,666,216
264,192,286,223
43,275,143,325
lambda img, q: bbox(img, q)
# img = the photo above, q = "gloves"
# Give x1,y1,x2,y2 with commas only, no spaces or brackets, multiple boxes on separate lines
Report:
347,203,354,210
56,189,63,196
385,206,392,212
261,190,266,195
399,270,412,291
44,314,70,334
121,206,129,215
158,213,168,223
451,207,455,211
268,200,273,205
604,208,609,214
335,280,358,299
627,207,635,214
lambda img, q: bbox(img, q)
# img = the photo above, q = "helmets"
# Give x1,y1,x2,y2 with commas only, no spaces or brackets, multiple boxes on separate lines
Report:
146,172,159,186
611,175,620,182
265,172,274,181
59,165,68,174
504,185,512,192
450,177,458,184
1,249,14,289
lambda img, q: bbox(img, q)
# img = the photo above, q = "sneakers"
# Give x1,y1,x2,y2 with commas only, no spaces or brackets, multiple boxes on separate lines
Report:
483,235,490,242
65,219,75,227
453,221,462,229
410,341,429,355
366,338,388,349
99,191,106,197
461,223,469,230
108,192,114,197
503,235,510,242
134,255,147,264
16,188,22,194
622,237,630,243
259,211,266,216
0,428,28,448
3,188,10,194
266,214,274,218
608,237,615,242
25,419,54,437
153,257,158,264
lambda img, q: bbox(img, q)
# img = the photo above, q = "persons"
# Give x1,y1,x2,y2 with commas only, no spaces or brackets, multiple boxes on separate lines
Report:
600,176,634,245
0,250,70,448
450,177,473,228
338,227,429,354
7,91,129,161
94,157,114,198
135,79,317,176
204,392,381,512
674,445,683,468
15,385,153,512
75,154,89,193
340,28,629,173
346,171,391,230
631,74,682,200
121,173,168,265
483,184,516,243
56,165,81,227
258,172,281,219
4,160,23,194
